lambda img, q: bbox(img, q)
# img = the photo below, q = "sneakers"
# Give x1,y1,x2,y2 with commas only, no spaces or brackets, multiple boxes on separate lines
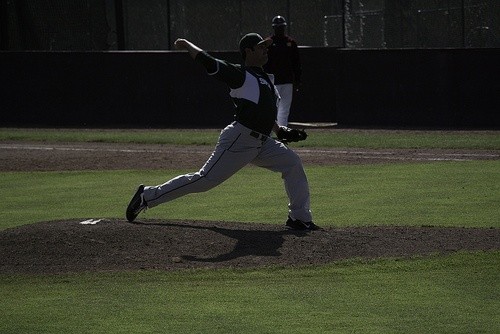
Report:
284,216,325,232
126,184,148,223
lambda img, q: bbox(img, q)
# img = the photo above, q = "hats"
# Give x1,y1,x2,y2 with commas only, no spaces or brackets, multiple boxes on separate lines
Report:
239,33,273,56
272,15,287,26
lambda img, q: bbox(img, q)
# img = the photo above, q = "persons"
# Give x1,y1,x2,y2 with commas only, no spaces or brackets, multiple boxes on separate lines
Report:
264,15,301,127
125,33,324,232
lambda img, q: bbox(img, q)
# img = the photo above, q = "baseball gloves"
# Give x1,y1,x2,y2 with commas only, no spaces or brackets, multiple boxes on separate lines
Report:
276,125,308,145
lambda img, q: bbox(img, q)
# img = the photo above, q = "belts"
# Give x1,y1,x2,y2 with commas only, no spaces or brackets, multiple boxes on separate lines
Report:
233,121,268,142
